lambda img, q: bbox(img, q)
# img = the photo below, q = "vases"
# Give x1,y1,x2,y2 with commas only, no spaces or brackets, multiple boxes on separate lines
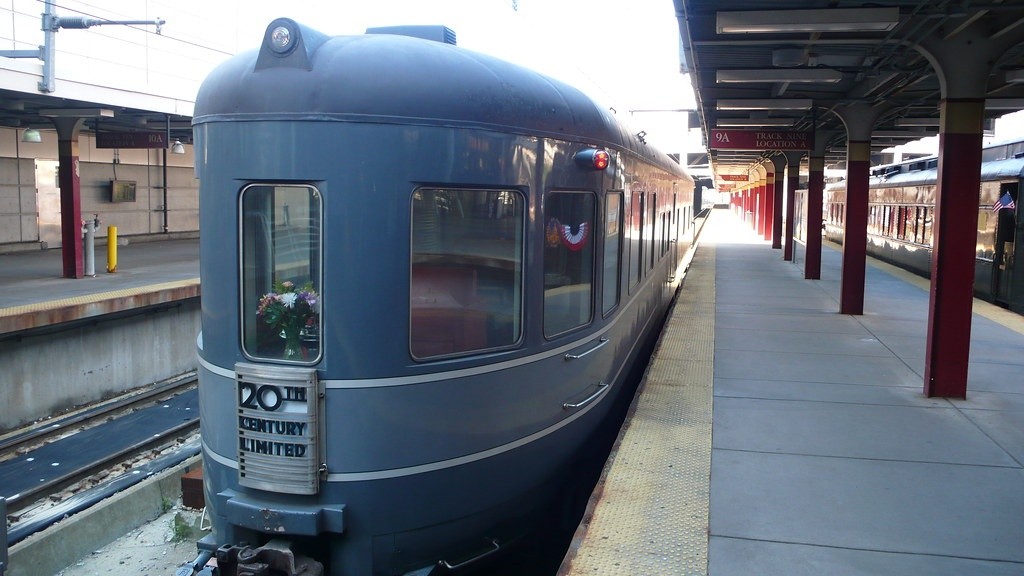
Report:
280,324,304,361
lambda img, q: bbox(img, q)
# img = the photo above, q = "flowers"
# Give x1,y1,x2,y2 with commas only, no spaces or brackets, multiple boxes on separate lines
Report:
256,278,320,329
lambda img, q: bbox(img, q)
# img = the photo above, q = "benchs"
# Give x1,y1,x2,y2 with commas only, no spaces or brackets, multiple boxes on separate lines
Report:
411,267,488,355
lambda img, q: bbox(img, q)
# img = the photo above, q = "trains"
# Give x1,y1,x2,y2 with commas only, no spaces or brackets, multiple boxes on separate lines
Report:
825,139,1024,318
173,18,693,576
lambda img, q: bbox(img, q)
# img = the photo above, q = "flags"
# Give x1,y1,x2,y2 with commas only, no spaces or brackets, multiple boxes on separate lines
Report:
992,192,1015,213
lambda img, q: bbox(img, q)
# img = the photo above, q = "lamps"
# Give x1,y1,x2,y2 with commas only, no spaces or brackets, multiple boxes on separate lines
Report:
715,6,900,35
169,137,186,154
19,127,43,143
715,69,842,84
144,122,193,130
716,119,794,127
715,99,812,110
716,149,766,165
38,108,114,118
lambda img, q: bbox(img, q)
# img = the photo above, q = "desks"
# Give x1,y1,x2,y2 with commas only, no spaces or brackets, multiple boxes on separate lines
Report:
449,238,514,270
